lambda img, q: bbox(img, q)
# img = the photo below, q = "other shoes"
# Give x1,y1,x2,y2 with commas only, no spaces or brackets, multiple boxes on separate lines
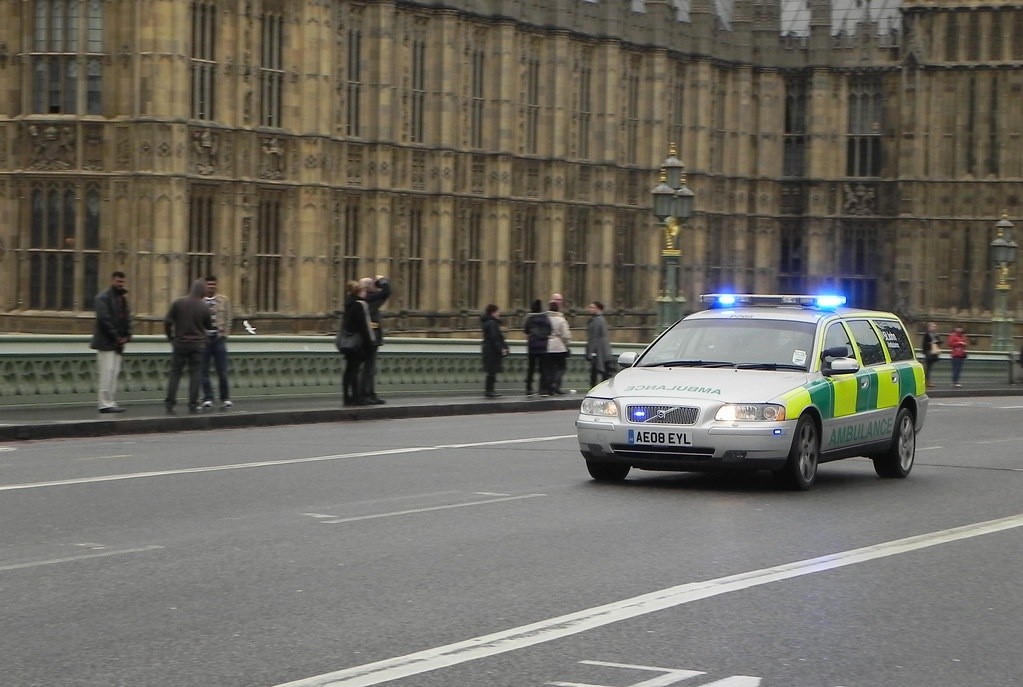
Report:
167,400,177,412
189,402,202,410
953,384,962,386
202,400,213,408
344,397,368,406
99,405,126,413
484,390,502,397
538,391,549,396
360,395,384,404
525,390,532,396
926,383,936,386
222,400,233,407
550,391,558,396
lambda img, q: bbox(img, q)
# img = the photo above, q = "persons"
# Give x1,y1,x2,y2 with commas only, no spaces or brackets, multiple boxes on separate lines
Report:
90,271,133,414
522,298,554,398
162,276,213,414
584,302,616,393
202,274,234,409
546,291,571,396
478,305,510,398
921,321,944,388
335,274,390,408
947,324,968,387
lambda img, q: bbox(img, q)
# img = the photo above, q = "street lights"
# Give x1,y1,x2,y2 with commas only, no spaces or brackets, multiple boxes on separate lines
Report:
652,143,696,335
990,209,1018,352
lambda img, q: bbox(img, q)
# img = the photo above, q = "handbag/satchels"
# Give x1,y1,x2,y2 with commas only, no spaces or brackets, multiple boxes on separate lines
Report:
931,343,941,355
336,329,364,354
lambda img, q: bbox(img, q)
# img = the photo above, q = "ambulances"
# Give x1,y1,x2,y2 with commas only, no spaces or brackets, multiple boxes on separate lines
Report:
575,294,930,491
